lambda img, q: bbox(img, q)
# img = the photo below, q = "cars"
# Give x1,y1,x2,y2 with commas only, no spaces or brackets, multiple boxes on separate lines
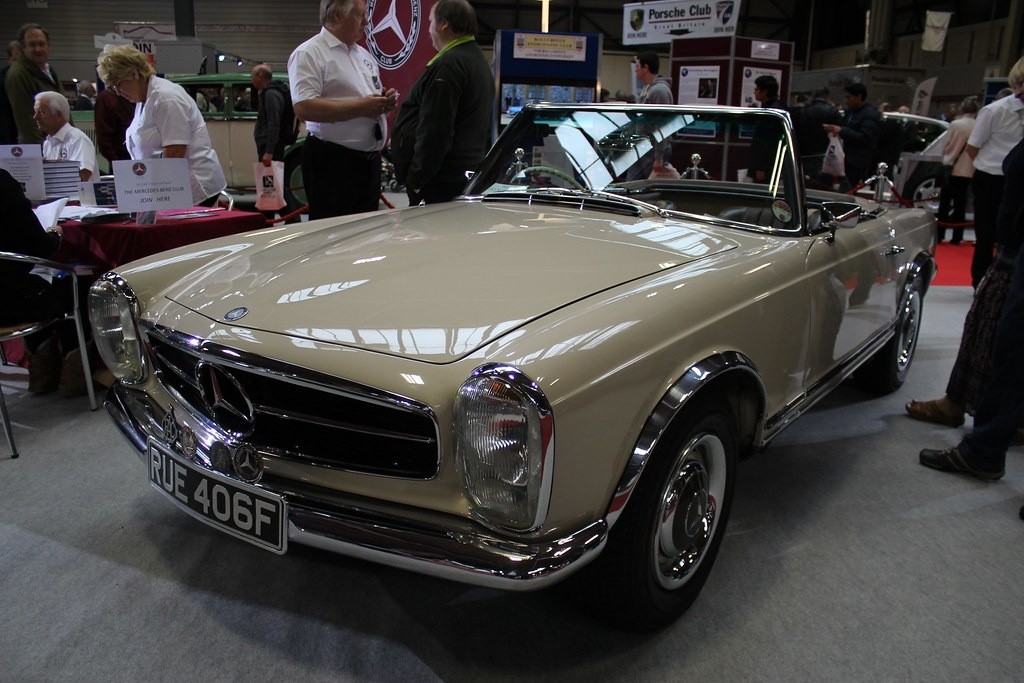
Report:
880,112,954,219
86,105,938,626
70,72,309,209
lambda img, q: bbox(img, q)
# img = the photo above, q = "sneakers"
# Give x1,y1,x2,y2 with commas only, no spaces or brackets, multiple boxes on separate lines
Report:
919,446,1005,481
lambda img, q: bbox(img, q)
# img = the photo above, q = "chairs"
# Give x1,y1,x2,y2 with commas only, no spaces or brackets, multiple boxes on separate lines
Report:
0,251,97,457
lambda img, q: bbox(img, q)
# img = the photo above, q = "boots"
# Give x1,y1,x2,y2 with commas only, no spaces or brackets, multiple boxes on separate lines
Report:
23,331,61,393
58,338,107,396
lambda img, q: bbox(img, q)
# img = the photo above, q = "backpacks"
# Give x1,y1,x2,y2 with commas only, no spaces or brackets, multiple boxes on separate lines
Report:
261,81,300,145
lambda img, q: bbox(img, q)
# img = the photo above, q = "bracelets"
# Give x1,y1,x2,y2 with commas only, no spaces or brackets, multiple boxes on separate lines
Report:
48,228,62,250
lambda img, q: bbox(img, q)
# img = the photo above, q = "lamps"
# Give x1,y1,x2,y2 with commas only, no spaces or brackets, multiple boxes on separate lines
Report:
217,52,225,62
236,59,242,66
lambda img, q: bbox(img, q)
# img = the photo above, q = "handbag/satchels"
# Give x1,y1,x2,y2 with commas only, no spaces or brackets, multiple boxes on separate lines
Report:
821,132,845,177
254,163,285,211
934,164,953,187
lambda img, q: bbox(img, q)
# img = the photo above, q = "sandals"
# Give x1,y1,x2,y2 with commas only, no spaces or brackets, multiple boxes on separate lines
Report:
906,399,965,428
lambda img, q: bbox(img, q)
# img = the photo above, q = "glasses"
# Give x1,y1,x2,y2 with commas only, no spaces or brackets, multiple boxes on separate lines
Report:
112,81,124,90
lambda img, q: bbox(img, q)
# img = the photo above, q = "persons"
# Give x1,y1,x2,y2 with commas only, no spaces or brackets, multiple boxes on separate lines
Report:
967,95,1024,287
194,87,253,111
601,88,609,101
747,75,788,184
904,136,1024,478
0,167,113,394
795,87,844,190
0,40,22,145
937,96,976,245
33,90,100,182
94,89,136,175
75,80,96,111
96,44,227,206
4,22,62,151
288,0,398,222
878,102,958,122
392,0,496,208
251,65,301,225
625,53,672,179
826,76,880,192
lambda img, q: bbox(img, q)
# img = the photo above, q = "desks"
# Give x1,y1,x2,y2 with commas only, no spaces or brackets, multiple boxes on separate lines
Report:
0,205,260,369
